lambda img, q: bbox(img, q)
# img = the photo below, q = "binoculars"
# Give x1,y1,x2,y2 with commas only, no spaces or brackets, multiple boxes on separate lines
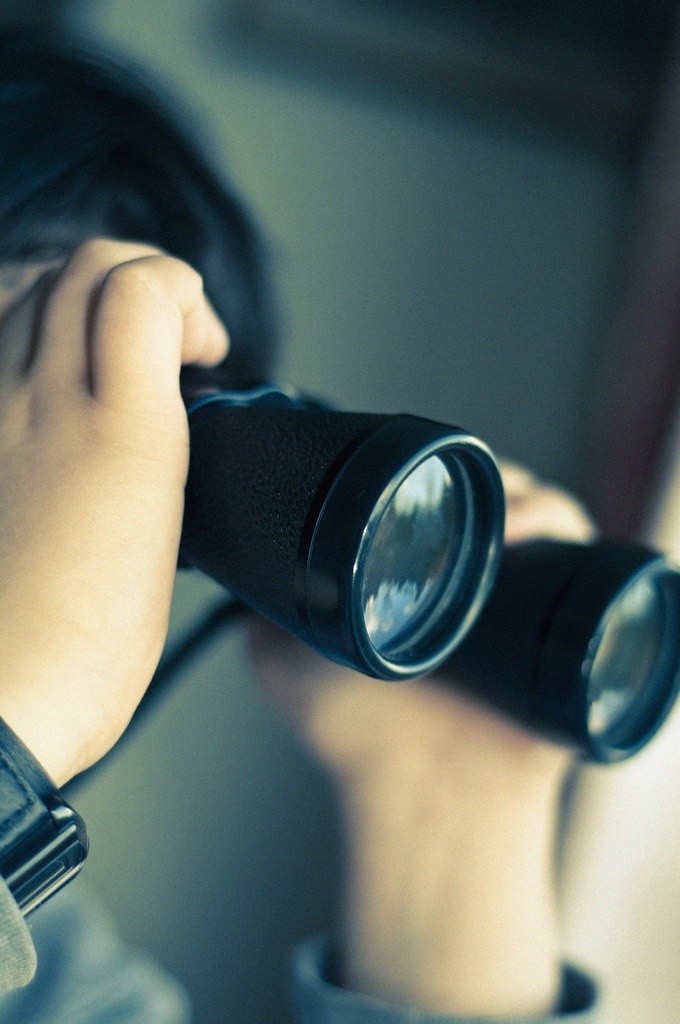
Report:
172,357,679,766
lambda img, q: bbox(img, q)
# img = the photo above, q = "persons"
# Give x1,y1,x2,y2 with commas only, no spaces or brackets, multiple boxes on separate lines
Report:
2,30,595,1024
